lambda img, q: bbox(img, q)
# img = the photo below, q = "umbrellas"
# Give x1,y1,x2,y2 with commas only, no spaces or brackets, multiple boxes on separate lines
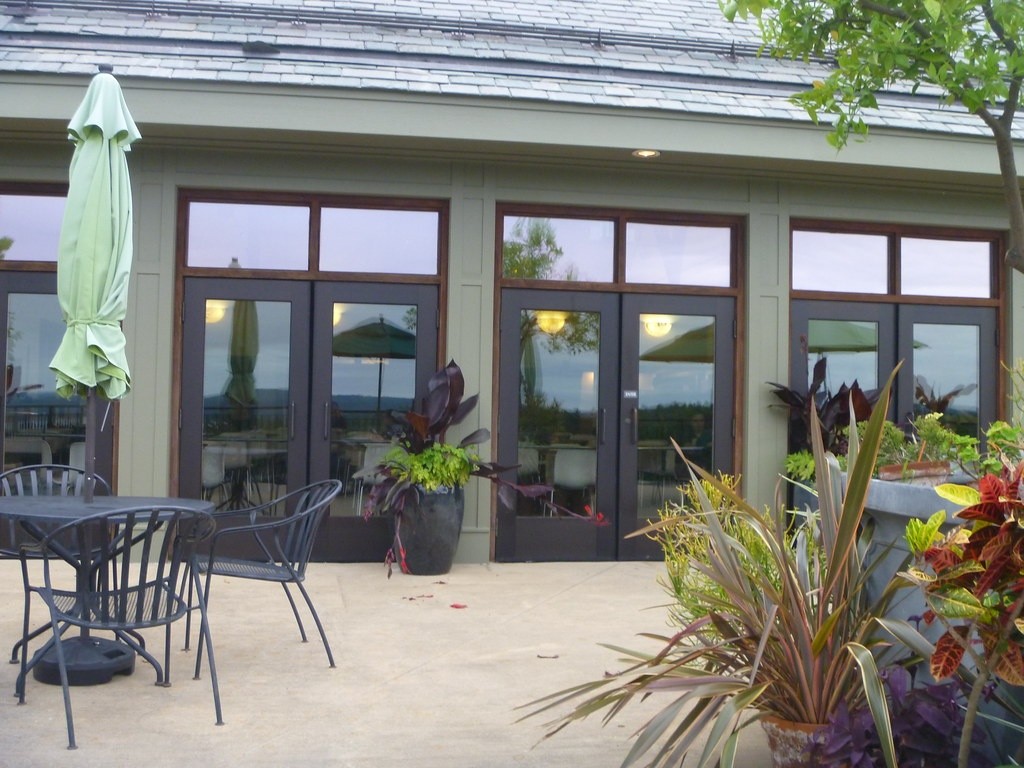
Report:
639,320,929,363
224,256,259,410
48,62,141,637
333,316,417,411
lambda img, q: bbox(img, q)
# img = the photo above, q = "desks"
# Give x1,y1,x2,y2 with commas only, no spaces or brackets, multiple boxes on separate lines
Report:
0,495,216,686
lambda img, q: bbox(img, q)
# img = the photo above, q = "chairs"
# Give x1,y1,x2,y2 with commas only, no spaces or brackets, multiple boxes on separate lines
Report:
0,464,344,747
67,442,596,517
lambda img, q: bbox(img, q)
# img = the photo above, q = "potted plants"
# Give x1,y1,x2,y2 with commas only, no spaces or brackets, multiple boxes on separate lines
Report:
835,412,981,488
509,359,1024,768
364,359,613,579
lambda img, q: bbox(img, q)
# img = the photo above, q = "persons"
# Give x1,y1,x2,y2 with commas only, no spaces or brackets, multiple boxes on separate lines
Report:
330,402,346,434
381,409,415,452
683,410,712,446
